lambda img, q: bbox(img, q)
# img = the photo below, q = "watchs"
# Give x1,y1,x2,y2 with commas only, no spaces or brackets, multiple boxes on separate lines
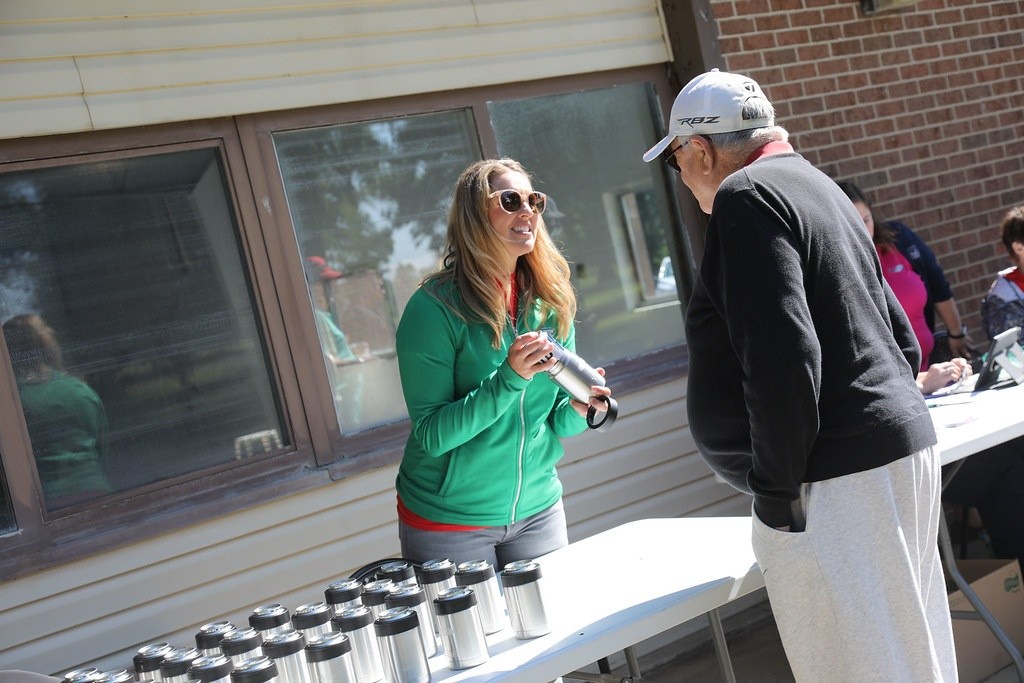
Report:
948,324,967,339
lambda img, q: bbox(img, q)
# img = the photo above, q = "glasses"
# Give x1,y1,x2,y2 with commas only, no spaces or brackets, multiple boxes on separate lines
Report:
663,142,691,172
488,189,547,215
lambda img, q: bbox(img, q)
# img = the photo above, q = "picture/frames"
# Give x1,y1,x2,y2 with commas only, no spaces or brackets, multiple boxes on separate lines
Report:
975,326,1024,391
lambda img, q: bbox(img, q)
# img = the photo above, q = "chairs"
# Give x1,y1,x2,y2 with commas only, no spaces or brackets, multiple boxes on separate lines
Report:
929,328,984,561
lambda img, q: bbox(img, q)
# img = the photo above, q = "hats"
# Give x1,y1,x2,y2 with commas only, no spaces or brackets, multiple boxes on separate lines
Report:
641,69,777,164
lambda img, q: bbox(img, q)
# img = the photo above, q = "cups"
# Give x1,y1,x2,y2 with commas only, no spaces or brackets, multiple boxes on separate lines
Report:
434,587,491,672
526,330,608,403
359,579,395,618
414,558,456,632
230,656,282,683
454,558,506,635
195,620,310,683
61,667,137,683
291,601,387,683
248,603,293,643
383,583,439,659
373,607,432,683
500,560,554,641
304,631,360,683
132,641,230,683
374,560,419,587
232,429,283,459
325,578,365,615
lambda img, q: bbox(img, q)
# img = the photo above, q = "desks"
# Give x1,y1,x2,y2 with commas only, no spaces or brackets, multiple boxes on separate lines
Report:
919,359,1024,683
343,516,765,683
335,347,409,435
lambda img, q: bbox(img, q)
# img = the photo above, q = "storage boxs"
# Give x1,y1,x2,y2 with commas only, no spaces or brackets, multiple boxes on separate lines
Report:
940,558,1024,683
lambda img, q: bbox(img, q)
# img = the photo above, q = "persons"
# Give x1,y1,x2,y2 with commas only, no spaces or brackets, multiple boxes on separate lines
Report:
5,312,111,508
836,179,975,373
910,358,967,394
641,66,960,683
394,159,611,572
316,311,368,433
981,206,1024,350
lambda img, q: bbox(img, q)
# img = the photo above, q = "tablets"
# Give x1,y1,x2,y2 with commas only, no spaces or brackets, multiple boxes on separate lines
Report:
974,326,1022,390
